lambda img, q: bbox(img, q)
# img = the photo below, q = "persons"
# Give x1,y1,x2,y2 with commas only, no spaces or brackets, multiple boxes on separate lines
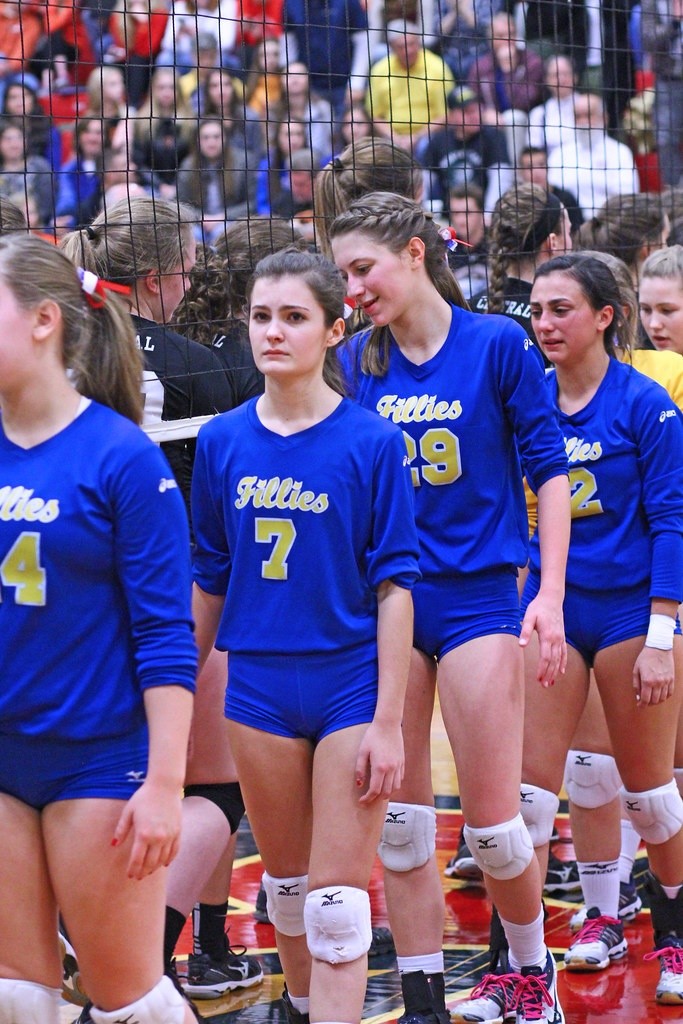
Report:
193,244,423,1024
0,231,199,1023
329,188,564,1024
452,253,682,1024
1,0,682,1024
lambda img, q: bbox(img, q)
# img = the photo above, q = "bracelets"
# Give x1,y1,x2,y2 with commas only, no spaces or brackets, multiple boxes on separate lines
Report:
645,613,677,650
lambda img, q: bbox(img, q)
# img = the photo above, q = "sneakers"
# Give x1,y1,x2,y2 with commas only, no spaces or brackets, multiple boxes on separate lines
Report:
397,1007,454,1024
454,973,520,1022
280,982,310,1024
444,844,484,882
252,879,272,923
564,907,628,971
570,872,643,933
507,948,565,1024
542,852,580,895
184,925,264,999
643,933,683,1005
56,914,92,1007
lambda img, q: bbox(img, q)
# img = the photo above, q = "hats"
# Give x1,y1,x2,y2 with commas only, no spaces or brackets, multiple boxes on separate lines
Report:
448,85,485,110
